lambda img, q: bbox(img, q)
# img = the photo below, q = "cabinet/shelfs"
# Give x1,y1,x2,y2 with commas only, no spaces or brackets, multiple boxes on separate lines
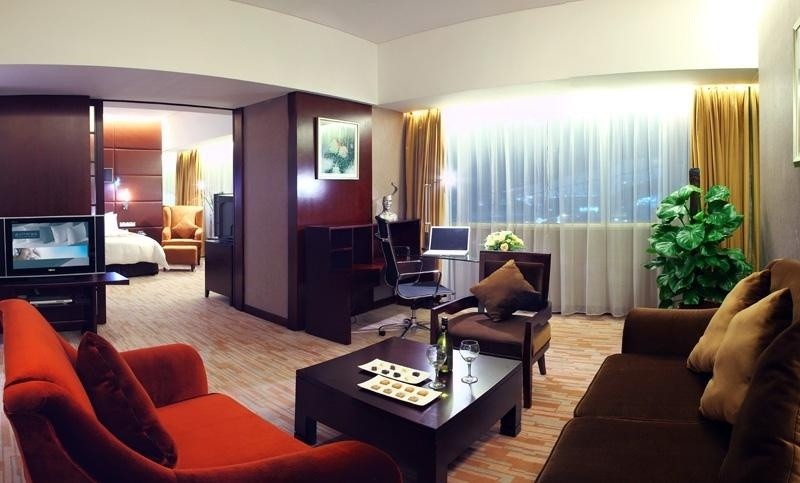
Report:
302,221,383,348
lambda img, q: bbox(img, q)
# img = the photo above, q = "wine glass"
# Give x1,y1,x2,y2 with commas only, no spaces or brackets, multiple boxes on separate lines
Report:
461,340,480,384
425,343,447,389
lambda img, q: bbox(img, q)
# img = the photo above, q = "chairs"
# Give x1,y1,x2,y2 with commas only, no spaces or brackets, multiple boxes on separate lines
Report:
161,205,205,267
374,216,456,339
430,251,552,409
1,298,404,483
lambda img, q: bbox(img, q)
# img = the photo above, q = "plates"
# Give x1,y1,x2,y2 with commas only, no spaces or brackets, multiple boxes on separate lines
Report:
357,357,432,385
357,374,442,407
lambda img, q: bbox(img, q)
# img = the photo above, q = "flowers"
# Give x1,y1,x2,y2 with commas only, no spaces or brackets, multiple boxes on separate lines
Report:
484,230,524,252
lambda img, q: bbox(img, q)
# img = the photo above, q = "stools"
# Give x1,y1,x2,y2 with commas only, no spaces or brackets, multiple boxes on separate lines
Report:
162,245,197,272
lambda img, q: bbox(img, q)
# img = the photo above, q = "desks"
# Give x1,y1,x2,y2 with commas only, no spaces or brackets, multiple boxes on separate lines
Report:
204,240,234,302
0,272,129,335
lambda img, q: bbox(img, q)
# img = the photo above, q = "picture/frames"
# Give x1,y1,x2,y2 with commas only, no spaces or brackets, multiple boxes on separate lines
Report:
791,16,800,164
313,115,360,182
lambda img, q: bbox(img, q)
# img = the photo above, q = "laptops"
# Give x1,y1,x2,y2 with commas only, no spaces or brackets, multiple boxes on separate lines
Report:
422,226,471,257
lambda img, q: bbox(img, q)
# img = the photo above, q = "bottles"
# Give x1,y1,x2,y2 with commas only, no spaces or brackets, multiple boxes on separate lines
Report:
438,316,453,373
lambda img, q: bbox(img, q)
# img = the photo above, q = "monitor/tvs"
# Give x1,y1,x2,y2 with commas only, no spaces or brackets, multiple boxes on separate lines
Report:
204,192,234,240
0,215,105,279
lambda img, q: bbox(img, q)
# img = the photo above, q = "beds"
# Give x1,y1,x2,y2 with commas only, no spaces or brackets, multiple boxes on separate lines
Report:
104,213,171,278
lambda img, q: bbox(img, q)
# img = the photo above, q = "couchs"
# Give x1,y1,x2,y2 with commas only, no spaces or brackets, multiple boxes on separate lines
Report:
536,258,800,482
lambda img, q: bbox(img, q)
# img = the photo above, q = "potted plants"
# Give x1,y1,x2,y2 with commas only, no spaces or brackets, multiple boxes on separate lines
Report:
642,184,754,309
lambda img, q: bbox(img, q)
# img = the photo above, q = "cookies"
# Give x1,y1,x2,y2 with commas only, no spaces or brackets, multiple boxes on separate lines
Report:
370,362,429,401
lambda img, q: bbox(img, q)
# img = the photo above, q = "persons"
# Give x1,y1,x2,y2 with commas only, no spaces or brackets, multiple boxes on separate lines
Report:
376,194,399,234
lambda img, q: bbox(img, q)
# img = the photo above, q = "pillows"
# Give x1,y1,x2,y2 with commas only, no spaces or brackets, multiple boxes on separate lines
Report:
105,211,114,215
76,325,178,470
469,259,541,323
172,215,199,239
698,288,793,423
685,268,772,372
105,215,119,236
720,321,800,482
